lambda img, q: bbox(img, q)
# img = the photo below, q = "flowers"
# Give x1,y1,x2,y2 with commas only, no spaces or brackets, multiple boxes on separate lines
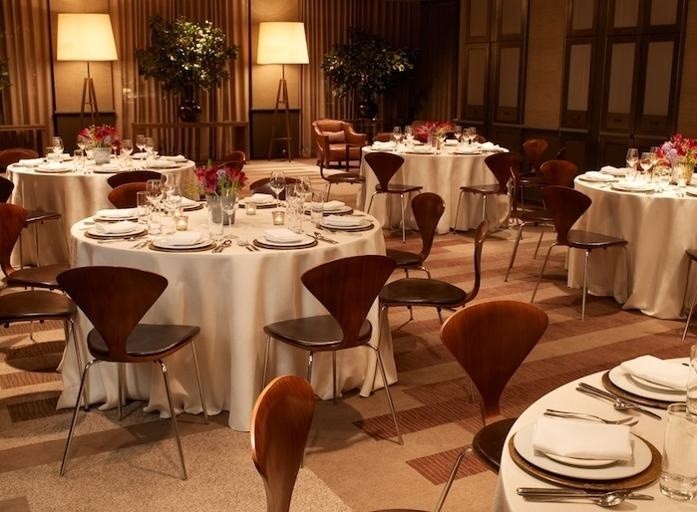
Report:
654,133,696,168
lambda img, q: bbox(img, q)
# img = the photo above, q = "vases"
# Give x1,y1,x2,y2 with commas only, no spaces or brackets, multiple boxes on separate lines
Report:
670,162,694,185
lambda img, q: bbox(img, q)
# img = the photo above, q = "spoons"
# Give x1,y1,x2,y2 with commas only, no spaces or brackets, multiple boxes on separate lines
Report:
515,488,638,507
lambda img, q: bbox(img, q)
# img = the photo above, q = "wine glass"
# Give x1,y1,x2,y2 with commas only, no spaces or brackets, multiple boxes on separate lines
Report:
625,144,691,190
122,134,155,163
269,172,327,233
134,172,184,231
390,123,478,149
46,134,66,166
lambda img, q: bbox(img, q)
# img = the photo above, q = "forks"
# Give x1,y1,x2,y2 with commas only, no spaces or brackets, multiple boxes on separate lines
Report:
542,407,640,428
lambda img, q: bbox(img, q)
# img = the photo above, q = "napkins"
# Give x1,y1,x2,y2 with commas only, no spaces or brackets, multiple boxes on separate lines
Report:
534,415,632,460
623,355,695,390
584,171,612,181
601,166,621,174
619,179,648,188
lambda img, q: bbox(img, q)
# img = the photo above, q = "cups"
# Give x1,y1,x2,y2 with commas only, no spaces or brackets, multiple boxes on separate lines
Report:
684,344,696,421
661,404,697,502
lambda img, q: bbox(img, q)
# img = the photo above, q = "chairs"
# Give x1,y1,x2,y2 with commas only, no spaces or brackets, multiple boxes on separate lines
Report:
531,184,629,322
436,298,549,512
680,249,696,342
2,140,446,512
505,160,577,281
319,121,547,244
370,220,490,402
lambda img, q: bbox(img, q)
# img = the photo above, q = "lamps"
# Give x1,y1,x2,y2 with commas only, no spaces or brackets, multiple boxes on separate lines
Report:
256,21,311,163
54,14,118,154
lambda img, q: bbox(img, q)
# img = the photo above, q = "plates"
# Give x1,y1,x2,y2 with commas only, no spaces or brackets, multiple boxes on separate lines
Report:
578,166,697,197
609,363,691,399
87,191,374,252
16,152,191,174
371,141,501,155
513,418,654,481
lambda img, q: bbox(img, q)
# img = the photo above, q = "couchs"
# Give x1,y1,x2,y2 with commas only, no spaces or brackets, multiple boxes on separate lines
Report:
311,121,366,168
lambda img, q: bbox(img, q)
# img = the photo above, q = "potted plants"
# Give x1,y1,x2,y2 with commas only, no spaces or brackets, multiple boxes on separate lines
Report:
317,24,418,119
130,16,241,122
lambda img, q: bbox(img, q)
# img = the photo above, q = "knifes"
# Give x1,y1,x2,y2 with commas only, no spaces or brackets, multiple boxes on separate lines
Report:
575,381,663,420
516,487,654,502
